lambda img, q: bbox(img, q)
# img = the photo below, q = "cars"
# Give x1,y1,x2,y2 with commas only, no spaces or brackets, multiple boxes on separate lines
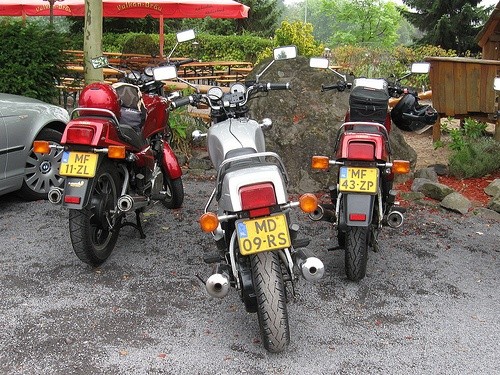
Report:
0,92,71,201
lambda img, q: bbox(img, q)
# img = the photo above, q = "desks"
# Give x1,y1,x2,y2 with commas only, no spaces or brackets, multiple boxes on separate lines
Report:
179,61,252,75
66,66,131,80
130,57,192,61
64,49,150,57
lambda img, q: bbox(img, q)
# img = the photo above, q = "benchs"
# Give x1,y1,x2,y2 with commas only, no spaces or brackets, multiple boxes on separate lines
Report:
53,53,253,108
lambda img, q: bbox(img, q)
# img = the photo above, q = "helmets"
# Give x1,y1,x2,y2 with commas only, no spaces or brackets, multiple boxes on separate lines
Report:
390,92,438,131
79,82,122,121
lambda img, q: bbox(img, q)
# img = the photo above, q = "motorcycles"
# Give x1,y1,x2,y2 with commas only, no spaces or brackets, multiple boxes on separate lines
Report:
307,55,431,281
152,45,325,353
59,27,196,267
